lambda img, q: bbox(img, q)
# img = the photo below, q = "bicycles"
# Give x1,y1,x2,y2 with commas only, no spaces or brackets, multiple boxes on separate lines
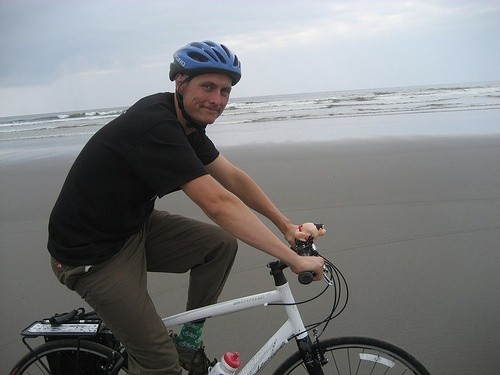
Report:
9,234,430,375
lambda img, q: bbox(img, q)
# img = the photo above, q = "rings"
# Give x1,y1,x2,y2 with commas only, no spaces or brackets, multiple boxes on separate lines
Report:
299,224,303,232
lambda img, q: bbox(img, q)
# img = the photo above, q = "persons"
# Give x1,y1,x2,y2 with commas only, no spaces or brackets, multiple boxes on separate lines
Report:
46,41,325,375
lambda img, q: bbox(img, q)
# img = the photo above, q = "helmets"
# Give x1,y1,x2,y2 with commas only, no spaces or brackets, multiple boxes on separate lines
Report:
169,41,243,86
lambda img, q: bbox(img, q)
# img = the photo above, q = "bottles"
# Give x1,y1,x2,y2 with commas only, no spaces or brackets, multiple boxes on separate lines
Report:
208,352,240,375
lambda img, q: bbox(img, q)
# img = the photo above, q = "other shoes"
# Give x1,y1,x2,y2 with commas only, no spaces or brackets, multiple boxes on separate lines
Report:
167,330,212,375
